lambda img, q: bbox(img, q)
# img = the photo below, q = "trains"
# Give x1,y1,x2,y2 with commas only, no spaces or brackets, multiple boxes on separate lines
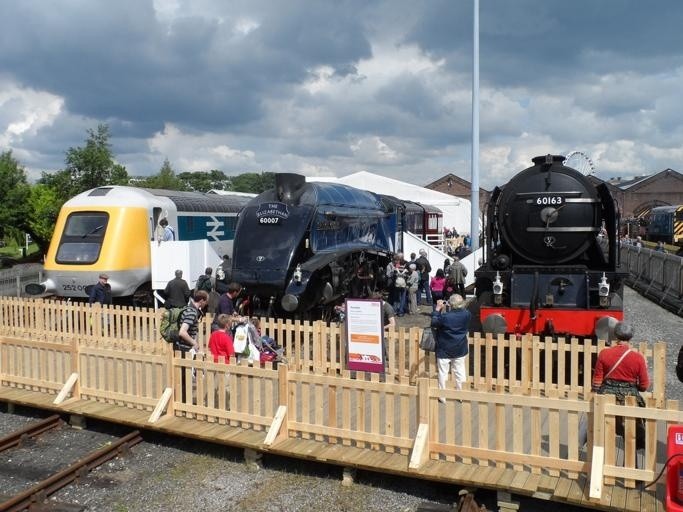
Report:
25,184,259,324
474,153,631,386
216,172,445,347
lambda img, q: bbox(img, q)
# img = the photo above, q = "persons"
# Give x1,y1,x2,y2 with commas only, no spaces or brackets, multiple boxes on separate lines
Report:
157,217,175,244
88,273,112,336
163,269,192,310
565,321,650,484
596,225,683,258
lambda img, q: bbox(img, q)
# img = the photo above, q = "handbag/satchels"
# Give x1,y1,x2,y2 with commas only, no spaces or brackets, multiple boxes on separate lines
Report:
232,325,252,358
578,411,588,446
419,327,437,352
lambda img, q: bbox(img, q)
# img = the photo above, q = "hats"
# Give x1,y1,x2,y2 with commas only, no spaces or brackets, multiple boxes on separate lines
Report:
99,274,110,280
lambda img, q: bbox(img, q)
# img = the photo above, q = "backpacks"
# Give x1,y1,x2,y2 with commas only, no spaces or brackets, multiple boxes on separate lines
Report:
394,269,407,288
194,275,213,295
159,306,198,343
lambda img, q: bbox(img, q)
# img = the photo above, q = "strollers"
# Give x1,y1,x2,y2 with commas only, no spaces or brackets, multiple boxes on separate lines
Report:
234,313,288,370
